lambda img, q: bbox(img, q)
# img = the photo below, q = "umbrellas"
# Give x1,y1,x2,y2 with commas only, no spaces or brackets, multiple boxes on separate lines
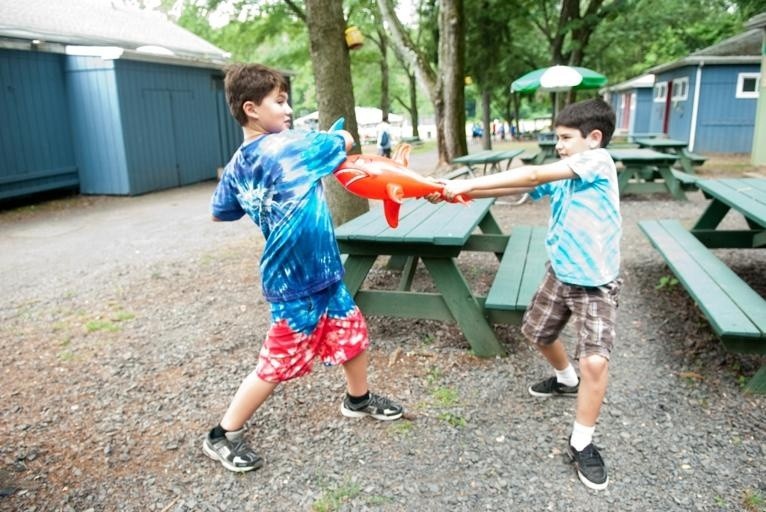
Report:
511,63,608,130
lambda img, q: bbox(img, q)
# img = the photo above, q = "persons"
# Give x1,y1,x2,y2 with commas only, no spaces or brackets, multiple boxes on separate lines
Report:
488,118,519,144
376,113,395,158
200,62,408,474
415,95,623,492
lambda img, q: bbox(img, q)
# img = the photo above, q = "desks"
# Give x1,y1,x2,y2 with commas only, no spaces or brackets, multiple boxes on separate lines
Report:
453,136,710,207
682,175,766,301
331,192,516,359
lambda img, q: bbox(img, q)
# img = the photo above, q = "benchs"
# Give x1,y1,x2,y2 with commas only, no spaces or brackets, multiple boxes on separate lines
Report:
636,215,766,396
479,221,553,326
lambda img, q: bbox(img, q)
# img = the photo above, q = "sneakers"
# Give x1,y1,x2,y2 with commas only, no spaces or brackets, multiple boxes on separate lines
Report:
339,390,403,420
567,433,609,491
529,376,582,399
202,425,264,474
